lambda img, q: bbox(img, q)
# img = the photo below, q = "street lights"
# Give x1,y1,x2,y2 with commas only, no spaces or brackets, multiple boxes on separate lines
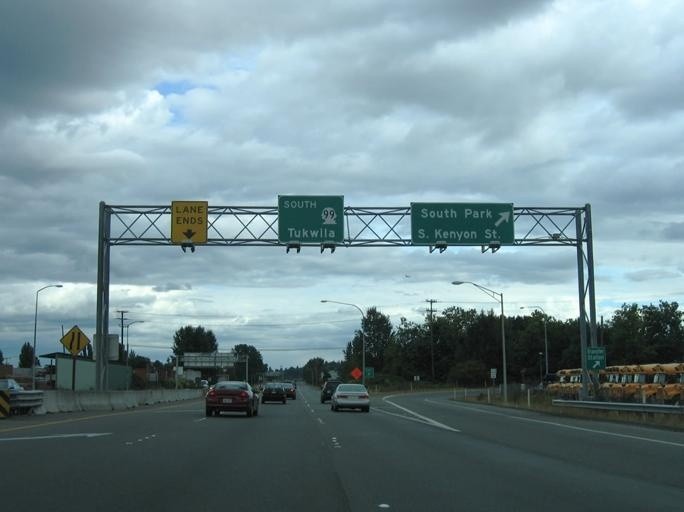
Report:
32,281,63,388
520,305,548,375
410,201,510,247
114,316,143,344
115,310,128,350
452,279,506,405
317,299,365,372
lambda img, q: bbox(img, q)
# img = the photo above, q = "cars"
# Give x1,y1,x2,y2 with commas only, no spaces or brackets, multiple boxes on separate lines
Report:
0,378,44,415
196,378,370,419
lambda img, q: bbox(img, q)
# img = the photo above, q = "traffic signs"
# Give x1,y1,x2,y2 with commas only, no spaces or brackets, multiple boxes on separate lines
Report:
586,346,607,373
